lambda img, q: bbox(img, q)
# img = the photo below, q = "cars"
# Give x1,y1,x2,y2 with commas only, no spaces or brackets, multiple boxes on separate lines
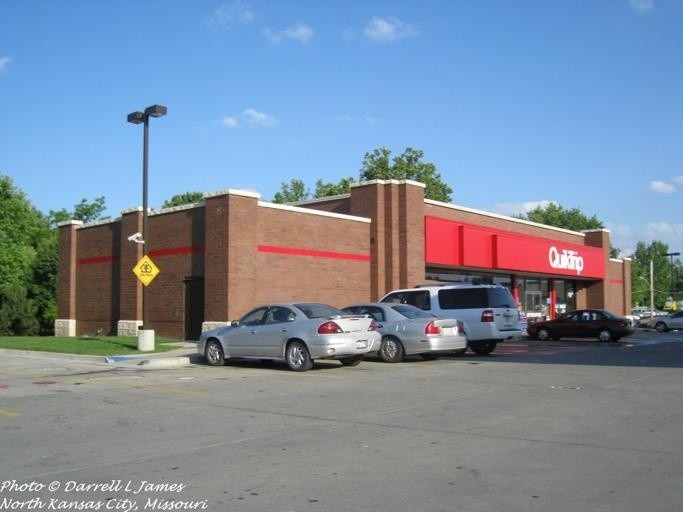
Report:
341,303,468,362
527,308,634,342
197,303,382,372
632,309,683,333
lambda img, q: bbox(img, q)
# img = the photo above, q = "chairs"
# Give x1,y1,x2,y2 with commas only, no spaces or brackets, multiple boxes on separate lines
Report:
582,315,587,321
274,309,285,321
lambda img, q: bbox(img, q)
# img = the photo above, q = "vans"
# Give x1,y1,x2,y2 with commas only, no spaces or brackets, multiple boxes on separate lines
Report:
377,284,524,355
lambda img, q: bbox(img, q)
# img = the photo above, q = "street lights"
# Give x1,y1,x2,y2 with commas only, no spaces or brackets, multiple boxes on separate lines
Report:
127,105,166,330
662,253,680,300
